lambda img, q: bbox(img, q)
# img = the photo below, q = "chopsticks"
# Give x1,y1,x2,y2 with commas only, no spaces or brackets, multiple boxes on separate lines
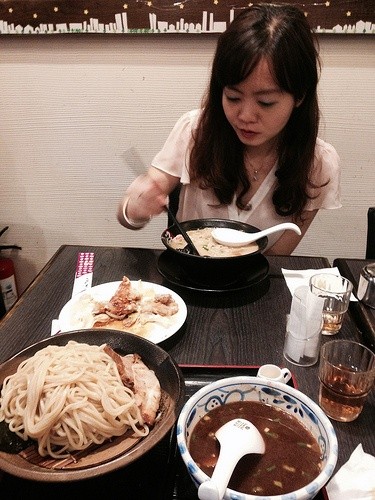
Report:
121,146,200,256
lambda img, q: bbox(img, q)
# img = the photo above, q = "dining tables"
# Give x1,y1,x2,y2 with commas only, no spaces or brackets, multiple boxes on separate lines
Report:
0,244,375,500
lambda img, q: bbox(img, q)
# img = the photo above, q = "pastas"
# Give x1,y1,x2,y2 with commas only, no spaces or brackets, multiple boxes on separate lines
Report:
0,340,151,457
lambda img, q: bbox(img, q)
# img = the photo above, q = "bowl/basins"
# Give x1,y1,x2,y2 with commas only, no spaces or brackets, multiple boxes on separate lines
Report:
177,376,338,500
161,218,268,283
0,328,185,481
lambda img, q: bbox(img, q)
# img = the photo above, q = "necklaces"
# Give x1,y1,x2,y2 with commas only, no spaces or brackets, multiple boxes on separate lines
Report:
245,143,275,181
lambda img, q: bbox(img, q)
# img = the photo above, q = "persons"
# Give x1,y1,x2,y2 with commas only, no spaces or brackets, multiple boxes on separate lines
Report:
117,2,343,255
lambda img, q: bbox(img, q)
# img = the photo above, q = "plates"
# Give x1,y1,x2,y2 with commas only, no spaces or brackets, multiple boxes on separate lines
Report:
58,281,187,344
155,249,271,293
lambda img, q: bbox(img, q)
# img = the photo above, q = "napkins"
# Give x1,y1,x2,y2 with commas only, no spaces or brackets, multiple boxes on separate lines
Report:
326,443,375,500
280,267,358,303
287,285,324,361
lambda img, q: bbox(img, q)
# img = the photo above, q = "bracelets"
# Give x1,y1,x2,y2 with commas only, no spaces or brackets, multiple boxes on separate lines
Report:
123,197,149,227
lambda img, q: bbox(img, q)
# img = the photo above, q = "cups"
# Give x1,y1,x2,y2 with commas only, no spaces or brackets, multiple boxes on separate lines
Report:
256,364,292,384
318,339,375,422
283,314,324,367
310,273,353,335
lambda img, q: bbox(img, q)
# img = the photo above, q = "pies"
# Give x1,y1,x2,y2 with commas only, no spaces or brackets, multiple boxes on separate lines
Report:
102,345,150,390
131,367,162,424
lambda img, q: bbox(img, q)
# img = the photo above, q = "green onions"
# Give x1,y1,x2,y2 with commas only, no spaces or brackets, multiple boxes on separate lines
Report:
192,407,318,493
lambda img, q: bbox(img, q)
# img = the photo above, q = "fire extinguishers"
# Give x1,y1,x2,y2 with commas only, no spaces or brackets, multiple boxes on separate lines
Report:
0,226,22,318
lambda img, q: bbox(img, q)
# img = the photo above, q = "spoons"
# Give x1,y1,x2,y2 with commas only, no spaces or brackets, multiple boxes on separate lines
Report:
212,222,302,247
198,418,265,500
